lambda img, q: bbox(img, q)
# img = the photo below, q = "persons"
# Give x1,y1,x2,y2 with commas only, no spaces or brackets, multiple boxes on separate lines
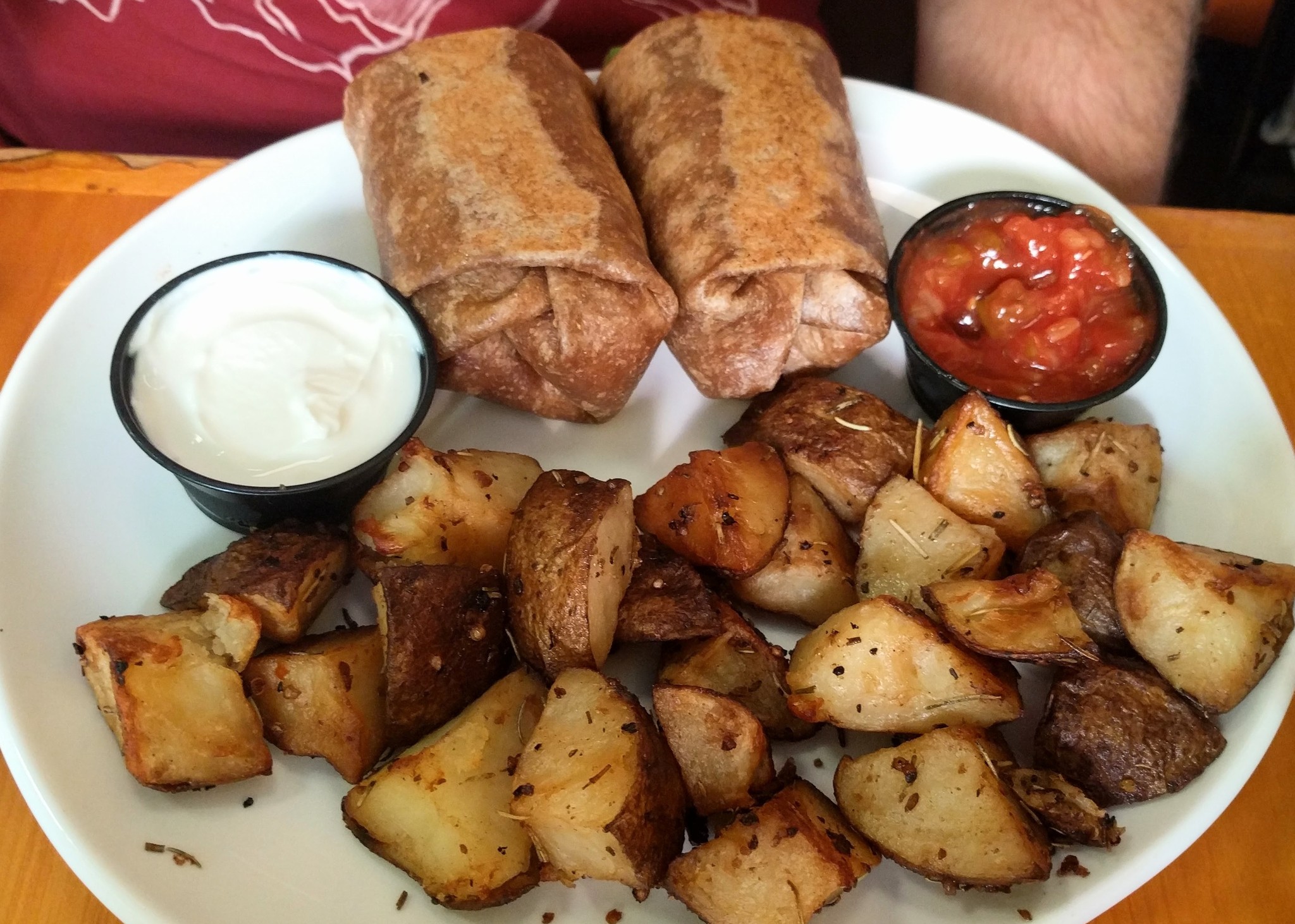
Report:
0,0,1198,207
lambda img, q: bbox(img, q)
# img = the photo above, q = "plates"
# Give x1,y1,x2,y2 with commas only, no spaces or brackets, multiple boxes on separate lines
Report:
1,67,1295,924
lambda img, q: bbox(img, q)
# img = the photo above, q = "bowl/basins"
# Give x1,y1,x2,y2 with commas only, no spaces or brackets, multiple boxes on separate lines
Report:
887,193,1168,440
108,249,432,537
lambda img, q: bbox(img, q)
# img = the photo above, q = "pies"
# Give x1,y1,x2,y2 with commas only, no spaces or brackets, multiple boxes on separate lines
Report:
593,9,893,399
345,24,680,425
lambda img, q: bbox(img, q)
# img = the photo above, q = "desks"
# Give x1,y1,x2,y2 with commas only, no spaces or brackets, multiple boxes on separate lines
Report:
0,149,1295,924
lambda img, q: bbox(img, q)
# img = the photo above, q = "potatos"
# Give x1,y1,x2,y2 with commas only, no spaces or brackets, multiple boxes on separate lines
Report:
71,373,1295,924
